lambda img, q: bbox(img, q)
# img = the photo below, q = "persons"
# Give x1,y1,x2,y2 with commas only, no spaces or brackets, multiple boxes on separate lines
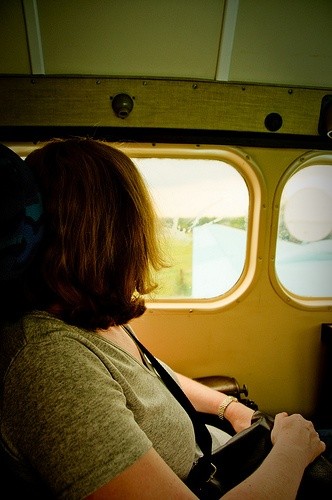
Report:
0,138,326,500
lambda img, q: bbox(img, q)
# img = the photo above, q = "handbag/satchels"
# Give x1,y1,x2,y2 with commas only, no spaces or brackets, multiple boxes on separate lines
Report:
121,324,331,500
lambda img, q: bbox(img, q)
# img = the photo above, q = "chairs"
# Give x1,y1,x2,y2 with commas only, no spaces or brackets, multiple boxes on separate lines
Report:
1,143,258,434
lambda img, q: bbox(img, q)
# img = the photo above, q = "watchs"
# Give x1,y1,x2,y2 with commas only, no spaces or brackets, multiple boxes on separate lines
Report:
218,395,238,420
